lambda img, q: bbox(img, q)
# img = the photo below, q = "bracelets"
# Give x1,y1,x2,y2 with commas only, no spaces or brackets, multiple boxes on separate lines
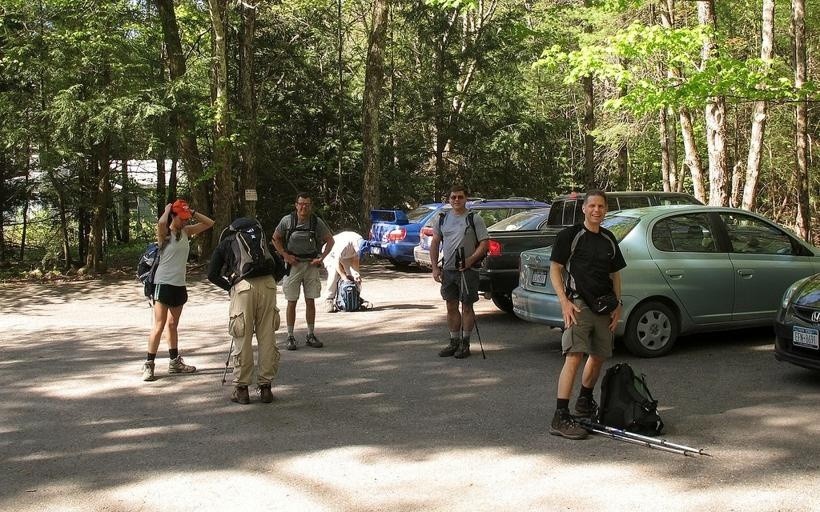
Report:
192,209,195,216
618,299,624,306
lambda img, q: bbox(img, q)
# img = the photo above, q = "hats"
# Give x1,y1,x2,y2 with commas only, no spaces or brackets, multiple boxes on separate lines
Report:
171,198,192,220
356,239,372,260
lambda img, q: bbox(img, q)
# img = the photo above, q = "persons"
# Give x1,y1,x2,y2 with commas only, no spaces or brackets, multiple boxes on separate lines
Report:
322,230,372,312
549,188,628,440
206,226,285,404
429,185,490,359
271,191,336,351
141,199,215,381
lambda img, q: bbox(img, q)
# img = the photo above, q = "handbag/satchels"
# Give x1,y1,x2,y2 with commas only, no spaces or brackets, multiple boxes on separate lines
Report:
574,264,618,316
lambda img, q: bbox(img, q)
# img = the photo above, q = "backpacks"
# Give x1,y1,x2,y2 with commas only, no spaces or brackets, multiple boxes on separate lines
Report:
137,235,171,307
219,214,275,284
335,274,364,311
599,364,664,437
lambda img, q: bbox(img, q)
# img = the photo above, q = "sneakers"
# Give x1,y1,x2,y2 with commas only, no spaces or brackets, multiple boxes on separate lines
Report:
304,334,323,348
168,355,195,372
258,384,273,403
230,385,250,404
285,336,297,350
438,338,461,357
549,409,588,439
143,359,154,382
575,395,598,417
455,338,471,358
325,299,334,313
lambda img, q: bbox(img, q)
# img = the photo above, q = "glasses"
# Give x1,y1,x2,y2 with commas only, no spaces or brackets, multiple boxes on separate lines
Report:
296,203,311,207
450,195,466,200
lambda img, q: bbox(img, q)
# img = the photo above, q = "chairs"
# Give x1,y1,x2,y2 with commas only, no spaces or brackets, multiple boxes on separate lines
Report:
684,226,706,251
